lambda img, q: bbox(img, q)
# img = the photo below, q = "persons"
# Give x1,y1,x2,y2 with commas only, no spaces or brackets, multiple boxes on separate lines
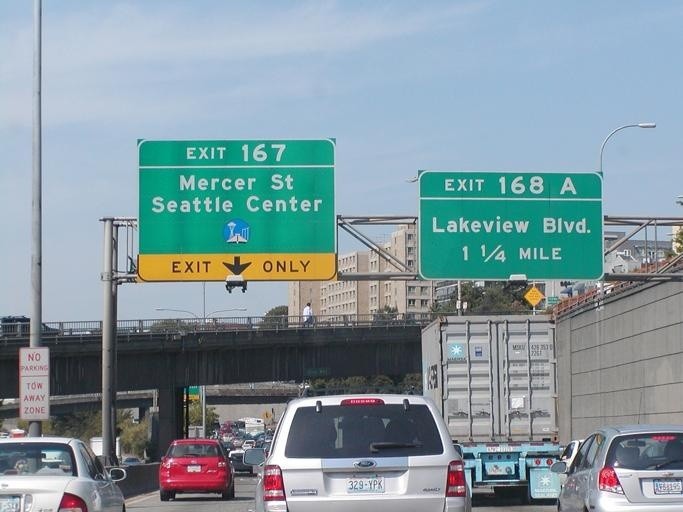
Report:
303,303,312,327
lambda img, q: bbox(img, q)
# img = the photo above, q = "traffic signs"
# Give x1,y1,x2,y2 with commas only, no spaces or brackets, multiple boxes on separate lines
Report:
416,169,607,283
137,136,337,285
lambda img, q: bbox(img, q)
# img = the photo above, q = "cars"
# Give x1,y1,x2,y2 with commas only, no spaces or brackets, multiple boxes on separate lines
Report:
240,392,474,510
553,423,683,512
122,457,143,466
158,437,237,502
0,437,129,512
210,415,275,474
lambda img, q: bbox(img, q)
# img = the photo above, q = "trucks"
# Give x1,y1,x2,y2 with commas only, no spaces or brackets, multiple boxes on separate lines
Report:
417,311,564,505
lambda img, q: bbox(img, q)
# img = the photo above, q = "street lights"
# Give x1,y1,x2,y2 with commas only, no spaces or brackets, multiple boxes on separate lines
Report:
596,121,659,291
